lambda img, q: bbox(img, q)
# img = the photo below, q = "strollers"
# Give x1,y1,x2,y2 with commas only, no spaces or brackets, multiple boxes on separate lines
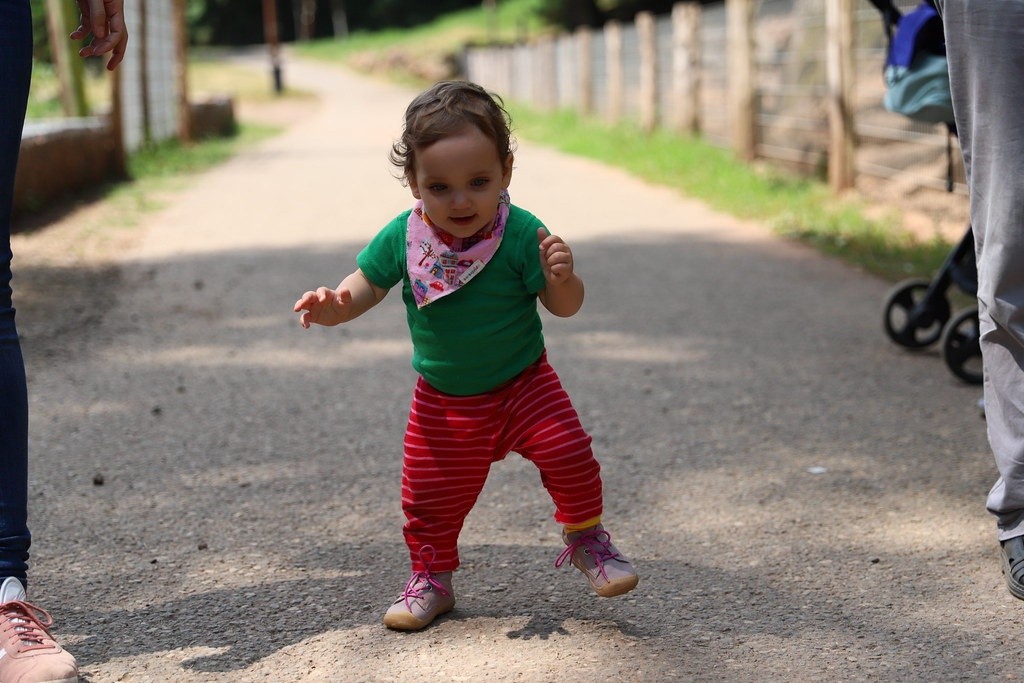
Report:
865,1,985,386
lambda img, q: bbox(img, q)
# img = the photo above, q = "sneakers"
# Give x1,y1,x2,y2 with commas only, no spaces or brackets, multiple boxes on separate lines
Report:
0,576,78,682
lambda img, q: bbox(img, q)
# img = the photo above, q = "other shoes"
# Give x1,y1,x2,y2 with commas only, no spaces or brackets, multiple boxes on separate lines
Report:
554,525,638,598
384,545,456,630
999,536,1024,599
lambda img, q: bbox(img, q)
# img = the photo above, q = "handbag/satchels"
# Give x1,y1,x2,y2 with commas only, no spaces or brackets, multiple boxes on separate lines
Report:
879,50,957,136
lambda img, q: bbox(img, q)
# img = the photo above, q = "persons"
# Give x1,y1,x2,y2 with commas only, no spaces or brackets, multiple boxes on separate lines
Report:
0,0,128,683
943,0,1024,599
295,80,639,632
